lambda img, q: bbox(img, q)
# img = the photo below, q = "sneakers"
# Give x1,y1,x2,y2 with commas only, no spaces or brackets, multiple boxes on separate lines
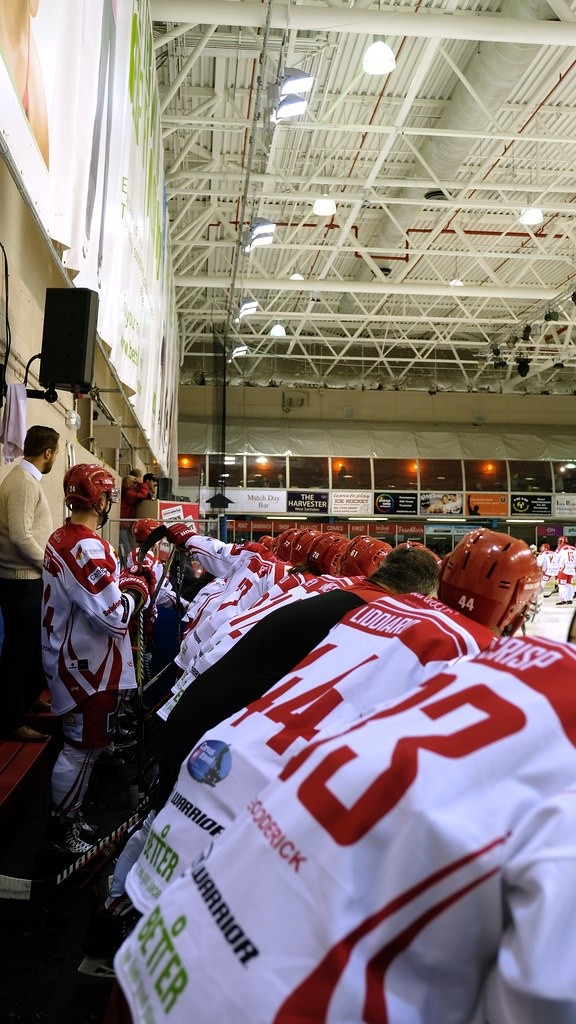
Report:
48,819,93,857
73,811,96,840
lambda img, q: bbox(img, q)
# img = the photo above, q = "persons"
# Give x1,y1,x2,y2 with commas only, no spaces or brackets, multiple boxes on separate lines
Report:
426,495,449,512
80,519,576,1023
0,426,61,741
443,494,461,514
39,465,157,855
120,469,157,552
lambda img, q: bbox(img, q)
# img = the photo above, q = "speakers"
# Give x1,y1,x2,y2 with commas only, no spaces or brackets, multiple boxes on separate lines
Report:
38,287,99,394
156,477,173,501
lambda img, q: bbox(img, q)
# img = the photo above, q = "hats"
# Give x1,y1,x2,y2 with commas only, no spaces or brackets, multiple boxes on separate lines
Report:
143,473,158,482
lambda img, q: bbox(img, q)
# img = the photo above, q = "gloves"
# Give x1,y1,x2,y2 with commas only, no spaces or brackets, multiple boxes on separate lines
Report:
119,566,157,615
168,524,198,551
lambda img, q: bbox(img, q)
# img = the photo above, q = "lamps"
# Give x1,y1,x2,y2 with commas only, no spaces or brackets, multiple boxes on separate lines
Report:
313,159,337,216
520,324,531,341
251,233,273,246
493,344,501,356
251,218,276,235
519,162,544,225
270,320,286,336
239,307,257,319
280,66,314,94
449,248,464,286
276,94,308,120
544,309,559,321
553,360,564,369
231,342,247,354
506,333,519,349
494,357,506,370
232,350,246,359
362,35,397,75
238,297,258,314
289,259,305,280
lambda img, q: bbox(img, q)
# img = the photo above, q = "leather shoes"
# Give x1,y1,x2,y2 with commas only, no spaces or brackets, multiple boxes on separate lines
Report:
15,726,50,742
29,699,52,711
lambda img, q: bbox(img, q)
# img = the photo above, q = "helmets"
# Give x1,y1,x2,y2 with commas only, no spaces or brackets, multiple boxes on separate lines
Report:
63,464,116,506
558,536,569,546
542,544,550,552
440,528,542,638
259,528,394,578
132,518,160,541
